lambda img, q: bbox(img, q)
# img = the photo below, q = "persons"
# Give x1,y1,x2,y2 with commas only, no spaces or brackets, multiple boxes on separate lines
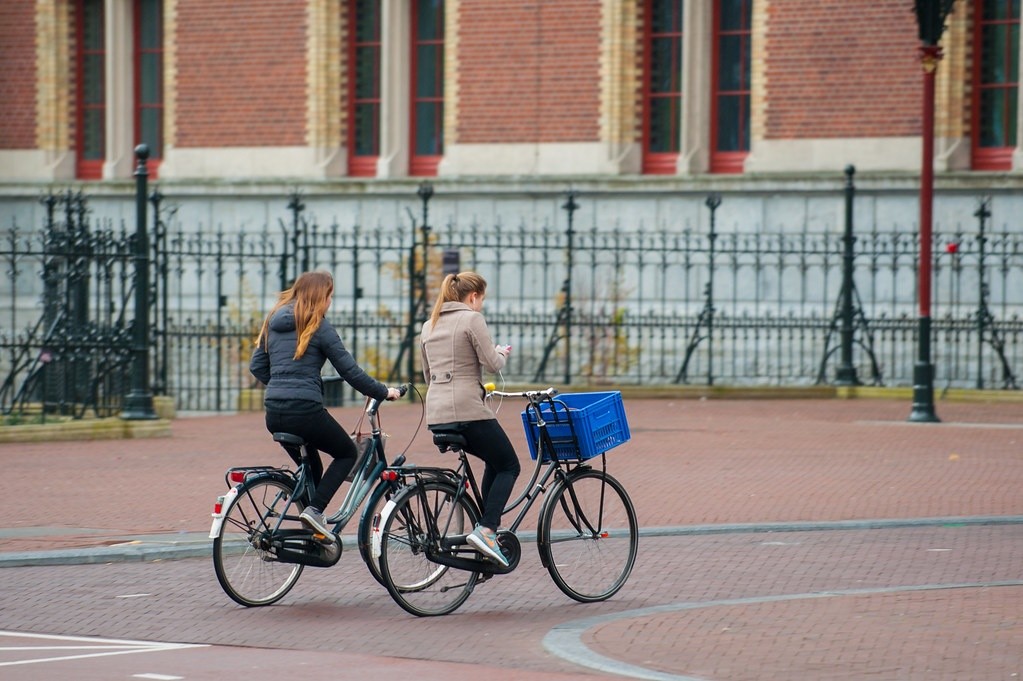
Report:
420,271,521,568
250,272,400,542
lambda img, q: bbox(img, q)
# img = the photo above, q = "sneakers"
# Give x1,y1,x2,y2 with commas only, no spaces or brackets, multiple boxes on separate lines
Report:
465,522,509,567
297,506,336,542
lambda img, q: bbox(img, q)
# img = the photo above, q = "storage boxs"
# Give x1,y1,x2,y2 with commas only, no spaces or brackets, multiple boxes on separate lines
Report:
520,390,630,463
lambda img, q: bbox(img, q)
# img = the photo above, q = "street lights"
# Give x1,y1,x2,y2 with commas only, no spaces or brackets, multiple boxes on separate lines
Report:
906,1,961,424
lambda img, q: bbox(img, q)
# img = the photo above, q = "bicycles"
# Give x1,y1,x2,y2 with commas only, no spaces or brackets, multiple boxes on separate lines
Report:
209,383,467,608
371,386,641,617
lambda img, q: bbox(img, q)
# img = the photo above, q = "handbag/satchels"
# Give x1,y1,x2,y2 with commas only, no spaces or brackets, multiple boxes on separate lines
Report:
344,396,387,479
